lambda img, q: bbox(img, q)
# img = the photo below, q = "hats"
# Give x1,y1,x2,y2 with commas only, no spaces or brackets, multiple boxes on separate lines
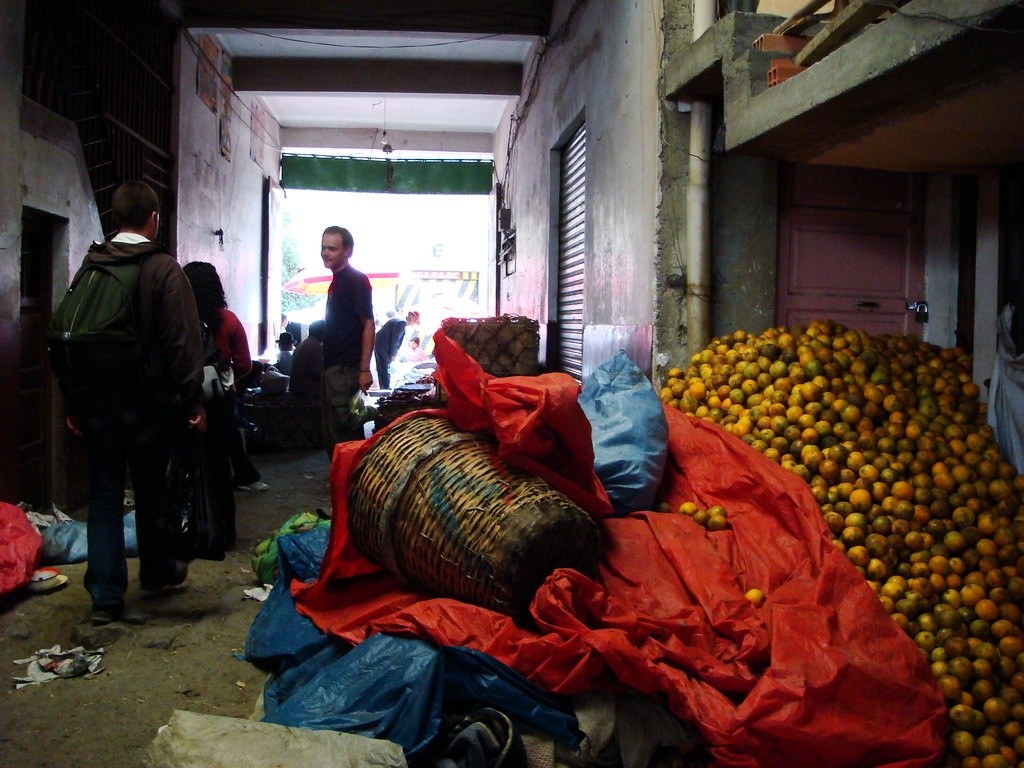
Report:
409,311,420,325
410,337,420,344
275,332,295,344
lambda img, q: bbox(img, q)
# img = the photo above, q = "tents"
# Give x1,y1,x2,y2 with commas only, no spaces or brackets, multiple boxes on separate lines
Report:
395,270,480,314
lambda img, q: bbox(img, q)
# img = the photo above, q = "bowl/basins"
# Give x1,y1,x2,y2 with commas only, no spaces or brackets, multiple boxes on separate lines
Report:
27,567,60,591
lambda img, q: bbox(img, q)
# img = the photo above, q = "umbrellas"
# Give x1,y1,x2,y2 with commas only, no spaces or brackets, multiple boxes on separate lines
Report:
283,260,401,297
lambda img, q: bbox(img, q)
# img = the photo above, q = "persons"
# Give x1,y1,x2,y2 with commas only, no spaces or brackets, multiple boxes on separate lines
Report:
317,225,376,519
181,261,270,491
375,306,430,390
234,312,325,435
46,179,210,623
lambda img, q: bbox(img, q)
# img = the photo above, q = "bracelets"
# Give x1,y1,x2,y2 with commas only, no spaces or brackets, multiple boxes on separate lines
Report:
360,370,371,373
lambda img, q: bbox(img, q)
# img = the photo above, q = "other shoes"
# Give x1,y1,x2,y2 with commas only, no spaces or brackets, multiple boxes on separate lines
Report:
145,559,187,595
238,480,270,493
91,603,122,623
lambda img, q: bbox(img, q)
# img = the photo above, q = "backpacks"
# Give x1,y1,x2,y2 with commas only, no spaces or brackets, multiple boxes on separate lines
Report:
199,318,230,403
42,251,152,416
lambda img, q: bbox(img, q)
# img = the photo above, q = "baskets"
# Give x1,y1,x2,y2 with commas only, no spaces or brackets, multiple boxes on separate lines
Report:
346,417,599,629
259,366,290,394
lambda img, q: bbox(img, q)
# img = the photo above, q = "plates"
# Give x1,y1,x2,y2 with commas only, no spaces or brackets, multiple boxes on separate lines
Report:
53,574,68,588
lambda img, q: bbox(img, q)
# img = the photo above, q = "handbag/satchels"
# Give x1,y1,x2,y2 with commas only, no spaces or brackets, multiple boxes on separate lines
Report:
346,387,379,429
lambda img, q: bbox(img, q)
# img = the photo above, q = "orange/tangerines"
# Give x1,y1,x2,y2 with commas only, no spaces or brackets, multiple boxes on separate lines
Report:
657,317,1024,768
